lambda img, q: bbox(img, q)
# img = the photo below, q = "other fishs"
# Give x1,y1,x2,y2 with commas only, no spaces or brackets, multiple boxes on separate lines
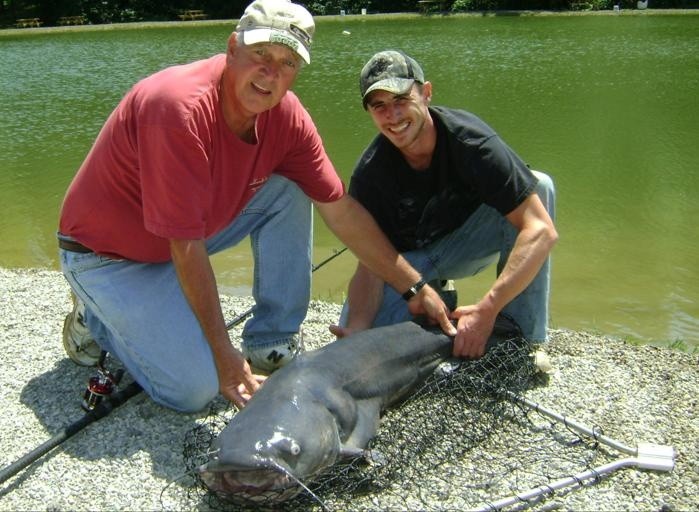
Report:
159,312,522,512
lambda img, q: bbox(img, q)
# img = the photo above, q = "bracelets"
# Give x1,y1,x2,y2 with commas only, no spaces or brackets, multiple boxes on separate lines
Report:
405,278,433,300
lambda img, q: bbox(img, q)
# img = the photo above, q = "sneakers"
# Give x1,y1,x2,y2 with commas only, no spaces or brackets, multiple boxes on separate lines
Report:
240,336,305,376
510,337,551,377
437,280,458,311
62,287,107,368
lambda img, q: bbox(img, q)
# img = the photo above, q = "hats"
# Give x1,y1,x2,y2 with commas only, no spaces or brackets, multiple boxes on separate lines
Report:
236,0,316,64
359,50,426,112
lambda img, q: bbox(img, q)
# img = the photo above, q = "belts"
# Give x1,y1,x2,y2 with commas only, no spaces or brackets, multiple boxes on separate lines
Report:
57,238,93,254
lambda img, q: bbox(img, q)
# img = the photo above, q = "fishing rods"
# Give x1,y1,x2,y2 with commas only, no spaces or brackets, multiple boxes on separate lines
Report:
1,247,347,480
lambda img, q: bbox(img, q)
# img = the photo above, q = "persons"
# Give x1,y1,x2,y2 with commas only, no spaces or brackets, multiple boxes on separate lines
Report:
58,1,460,412
331,49,563,376
